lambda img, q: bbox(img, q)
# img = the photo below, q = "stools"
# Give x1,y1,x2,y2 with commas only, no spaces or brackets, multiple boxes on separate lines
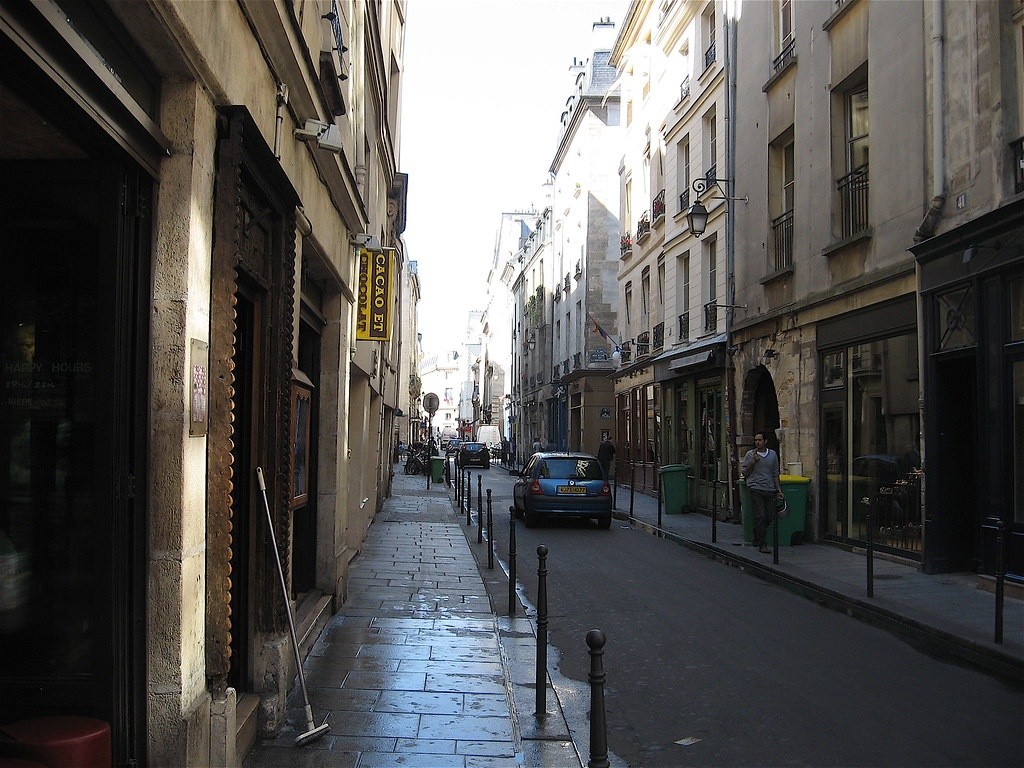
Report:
0,715,112,768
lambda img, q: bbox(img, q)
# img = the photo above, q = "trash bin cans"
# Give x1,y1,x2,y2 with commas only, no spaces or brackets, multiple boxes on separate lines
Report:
738,475,812,546
0,716,111,768
430,456,446,482
658,464,695,514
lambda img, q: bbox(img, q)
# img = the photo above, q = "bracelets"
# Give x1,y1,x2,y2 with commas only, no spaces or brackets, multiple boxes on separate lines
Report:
777,489,782,493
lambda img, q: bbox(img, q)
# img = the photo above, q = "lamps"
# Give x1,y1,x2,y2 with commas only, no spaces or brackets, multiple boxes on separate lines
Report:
527,323,550,352
631,338,652,346
604,354,622,361
293,118,342,155
685,177,729,238
350,232,381,253
615,346,635,353
707,304,748,315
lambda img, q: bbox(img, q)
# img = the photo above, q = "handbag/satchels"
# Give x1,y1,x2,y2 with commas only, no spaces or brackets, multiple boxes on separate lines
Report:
539,441,543,452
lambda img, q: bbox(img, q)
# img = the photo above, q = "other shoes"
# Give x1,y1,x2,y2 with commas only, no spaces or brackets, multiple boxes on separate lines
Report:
759,543,772,553
752,540,758,547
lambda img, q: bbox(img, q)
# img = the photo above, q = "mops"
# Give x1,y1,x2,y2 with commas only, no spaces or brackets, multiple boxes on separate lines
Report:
256,466,332,747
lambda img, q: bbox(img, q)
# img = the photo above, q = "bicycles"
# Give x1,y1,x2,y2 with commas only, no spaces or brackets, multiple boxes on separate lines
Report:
403,442,447,475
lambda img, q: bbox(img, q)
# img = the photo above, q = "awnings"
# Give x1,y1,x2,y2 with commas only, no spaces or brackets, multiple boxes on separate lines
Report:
668,350,713,371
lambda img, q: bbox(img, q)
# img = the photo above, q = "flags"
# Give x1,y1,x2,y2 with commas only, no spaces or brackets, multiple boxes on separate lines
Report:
592,320,607,341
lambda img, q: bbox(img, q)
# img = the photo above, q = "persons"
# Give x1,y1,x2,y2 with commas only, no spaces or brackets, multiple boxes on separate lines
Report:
499,437,509,467
559,437,567,452
741,431,785,553
474,435,477,442
450,435,461,439
509,438,515,468
399,441,406,458
466,435,471,441
597,437,615,481
532,438,542,452
546,439,554,451
420,432,441,454
488,442,501,465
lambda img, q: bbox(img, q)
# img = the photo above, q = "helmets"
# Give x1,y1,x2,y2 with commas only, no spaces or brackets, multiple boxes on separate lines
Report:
777,498,790,519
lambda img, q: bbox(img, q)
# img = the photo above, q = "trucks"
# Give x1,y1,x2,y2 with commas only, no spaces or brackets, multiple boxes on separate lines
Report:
440,436,450,450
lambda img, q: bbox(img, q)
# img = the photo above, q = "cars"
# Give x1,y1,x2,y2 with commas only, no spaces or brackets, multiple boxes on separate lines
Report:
453,441,491,470
509,451,612,530
446,439,463,455
840,453,899,490
